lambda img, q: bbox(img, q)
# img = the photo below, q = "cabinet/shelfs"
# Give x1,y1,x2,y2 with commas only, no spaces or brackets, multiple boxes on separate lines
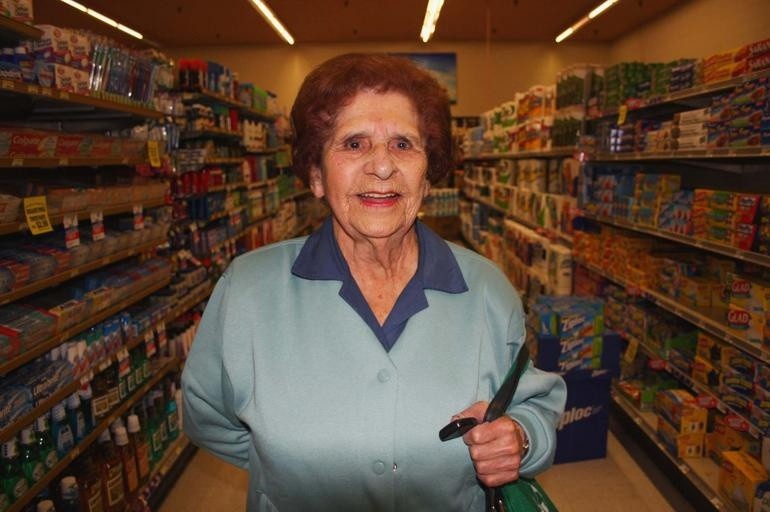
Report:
0,10,333,510
456,67,769,512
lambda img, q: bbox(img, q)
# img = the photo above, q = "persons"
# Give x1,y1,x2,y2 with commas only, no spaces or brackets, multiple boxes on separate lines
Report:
180,49,570,512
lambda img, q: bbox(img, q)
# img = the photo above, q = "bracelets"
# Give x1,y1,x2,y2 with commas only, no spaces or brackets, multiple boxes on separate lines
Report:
523,433,531,452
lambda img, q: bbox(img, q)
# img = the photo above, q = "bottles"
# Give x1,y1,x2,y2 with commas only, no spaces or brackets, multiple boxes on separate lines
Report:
0,387,93,512
93,345,178,512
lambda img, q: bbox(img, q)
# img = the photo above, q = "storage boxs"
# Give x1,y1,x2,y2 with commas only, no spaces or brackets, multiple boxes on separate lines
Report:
421,216,460,239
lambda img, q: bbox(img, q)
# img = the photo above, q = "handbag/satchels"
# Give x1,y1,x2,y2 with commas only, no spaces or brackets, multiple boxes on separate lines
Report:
500,476,560,512
